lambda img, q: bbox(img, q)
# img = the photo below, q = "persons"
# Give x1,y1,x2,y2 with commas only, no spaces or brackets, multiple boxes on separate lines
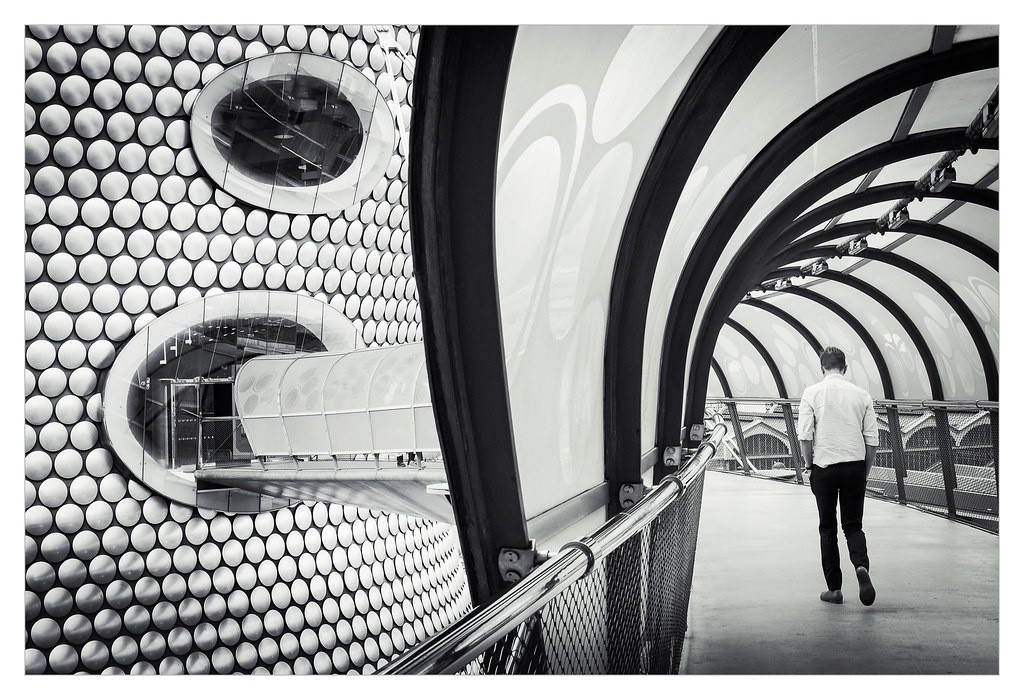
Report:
396,452,426,468
796,346,879,606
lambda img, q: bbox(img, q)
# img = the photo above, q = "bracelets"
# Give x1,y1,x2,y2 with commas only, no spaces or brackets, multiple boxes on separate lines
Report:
805,464,812,470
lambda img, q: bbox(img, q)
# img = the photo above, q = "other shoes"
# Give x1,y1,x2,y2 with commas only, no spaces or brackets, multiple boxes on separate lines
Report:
820,590,843,604
856,566,876,606
